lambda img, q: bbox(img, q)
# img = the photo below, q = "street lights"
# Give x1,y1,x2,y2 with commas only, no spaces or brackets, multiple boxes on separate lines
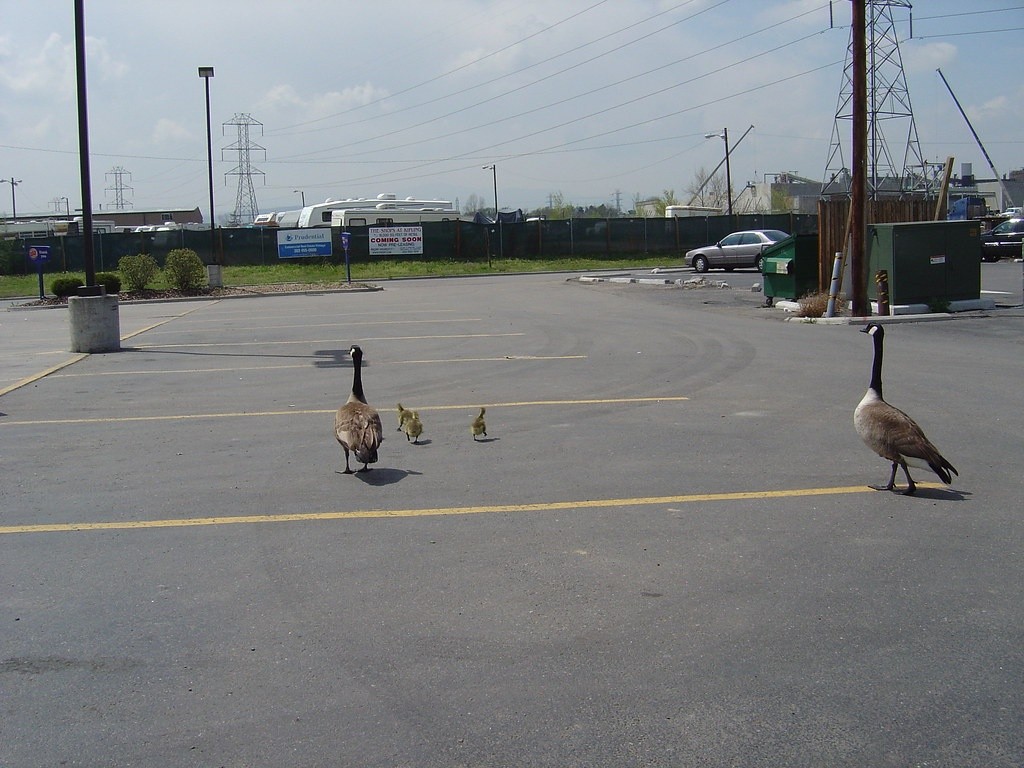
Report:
293,189,305,208
61,196,70,222
197,65,221,286
11,177,22,223
705,127,734,216
481,163,501,214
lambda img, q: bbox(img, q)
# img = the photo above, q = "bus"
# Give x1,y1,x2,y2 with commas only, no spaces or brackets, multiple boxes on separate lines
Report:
252,193,464,227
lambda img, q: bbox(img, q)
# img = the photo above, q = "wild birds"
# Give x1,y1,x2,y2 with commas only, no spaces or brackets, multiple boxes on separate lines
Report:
334,344,384,474
396,402,424,445
469,407,487,441
852,321,959,496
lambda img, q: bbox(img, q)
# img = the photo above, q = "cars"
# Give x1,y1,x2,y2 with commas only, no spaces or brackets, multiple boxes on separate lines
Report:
980,216,1024,263
684,229,792,273
1000,207,1024,219
